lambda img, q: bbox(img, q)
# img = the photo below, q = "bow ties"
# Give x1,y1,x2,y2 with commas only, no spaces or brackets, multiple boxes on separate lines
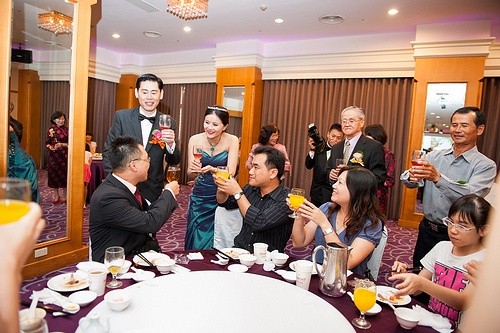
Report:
138,114,155,123
326,147,331,151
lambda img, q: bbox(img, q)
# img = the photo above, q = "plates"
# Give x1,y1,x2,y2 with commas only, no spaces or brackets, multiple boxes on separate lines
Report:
218,248,250,260
289,260,322,274
47,273,89,292
368,286,411,305
133,252,170,266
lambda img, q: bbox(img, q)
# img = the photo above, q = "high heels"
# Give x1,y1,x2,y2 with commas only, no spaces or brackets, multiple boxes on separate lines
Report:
60,200,66,204
54,196,60,204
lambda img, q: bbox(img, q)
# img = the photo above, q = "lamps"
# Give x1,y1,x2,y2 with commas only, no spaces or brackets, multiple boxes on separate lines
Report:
37,11,72,34
441,94,446,110
167,0,209,21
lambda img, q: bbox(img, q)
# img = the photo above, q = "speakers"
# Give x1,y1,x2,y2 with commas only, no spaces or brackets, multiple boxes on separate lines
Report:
12,48,32,63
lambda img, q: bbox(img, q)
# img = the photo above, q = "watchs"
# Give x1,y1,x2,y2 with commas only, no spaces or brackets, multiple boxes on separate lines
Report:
233,191,245,201
322,226,335,236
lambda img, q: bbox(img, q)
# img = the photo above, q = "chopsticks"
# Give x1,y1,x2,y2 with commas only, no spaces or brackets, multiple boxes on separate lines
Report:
134,250,154,266
211,247,234,260
20,301,71,315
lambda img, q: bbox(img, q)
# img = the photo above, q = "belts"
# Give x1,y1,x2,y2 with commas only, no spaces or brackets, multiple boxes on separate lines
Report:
428,222,448,234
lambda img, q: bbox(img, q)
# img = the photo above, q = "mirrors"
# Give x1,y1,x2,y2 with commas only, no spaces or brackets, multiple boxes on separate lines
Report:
413,81,468,215
4,0,78,248
222,85,245,185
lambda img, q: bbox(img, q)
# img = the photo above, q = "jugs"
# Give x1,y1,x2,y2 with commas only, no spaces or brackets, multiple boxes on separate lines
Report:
312,242,353,297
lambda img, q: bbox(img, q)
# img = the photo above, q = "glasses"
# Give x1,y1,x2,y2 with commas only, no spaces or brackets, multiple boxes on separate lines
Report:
442,217,481,232
341,119,360,124
128,157,150,167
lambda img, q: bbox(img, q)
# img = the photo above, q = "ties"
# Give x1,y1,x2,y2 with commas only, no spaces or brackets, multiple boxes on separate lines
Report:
134,188,142,206
344,141,350,165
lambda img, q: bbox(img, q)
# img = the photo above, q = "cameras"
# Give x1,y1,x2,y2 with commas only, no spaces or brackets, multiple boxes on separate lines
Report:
307,123,325,153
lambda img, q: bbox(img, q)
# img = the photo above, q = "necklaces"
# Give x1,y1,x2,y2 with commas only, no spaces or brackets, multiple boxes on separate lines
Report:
207,135,221,157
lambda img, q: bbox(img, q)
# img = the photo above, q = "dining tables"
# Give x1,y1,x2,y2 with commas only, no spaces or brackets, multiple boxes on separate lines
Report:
19,250,458,333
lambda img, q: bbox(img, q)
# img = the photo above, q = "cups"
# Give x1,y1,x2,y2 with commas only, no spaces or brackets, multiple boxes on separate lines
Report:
159,115,171,129
411,150,426,166
0,178,30,226
193,145,203,161
216,166,229,182
253,243,268,264
336,159,346,169
88,268,107,296
296,264,313,291
166,166,180,183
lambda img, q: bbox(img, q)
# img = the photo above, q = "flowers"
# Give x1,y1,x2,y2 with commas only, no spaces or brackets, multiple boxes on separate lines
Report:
149,130,165,149
350,150,366,167
49,128,55,138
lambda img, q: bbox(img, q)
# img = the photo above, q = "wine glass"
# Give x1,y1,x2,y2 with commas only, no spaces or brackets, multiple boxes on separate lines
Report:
104,247,125,288
288,188,305,218
352,282,376,329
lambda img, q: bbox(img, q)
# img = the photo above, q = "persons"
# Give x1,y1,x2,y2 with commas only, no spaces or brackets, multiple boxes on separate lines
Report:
187,173,198,187
212,144,297,254
88,136,180,264
7,117,40,205
9,101,23,144
324,106,387,202
185,105,239,250
83,143,93,209
400,106,497,308
286,165,386,282
244,123,291,185
45,110,69,203
364,123,396,214
481,169,500,247
0,202,46,333
387,193,495,333
456,168,500,333
102,73,182,238
462,259,483,288
305,123,345,209
86,130,97,154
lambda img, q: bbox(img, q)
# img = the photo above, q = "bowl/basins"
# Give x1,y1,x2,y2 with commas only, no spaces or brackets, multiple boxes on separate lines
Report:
19,308,46,331
271,253,289,266
282,271,296,283
228,264,248,273
69,291,97,306
104,289,132,311
239,254,257,268
117,260,132,274
394,307,420,329
365,303,382,315
155,259,175,274
